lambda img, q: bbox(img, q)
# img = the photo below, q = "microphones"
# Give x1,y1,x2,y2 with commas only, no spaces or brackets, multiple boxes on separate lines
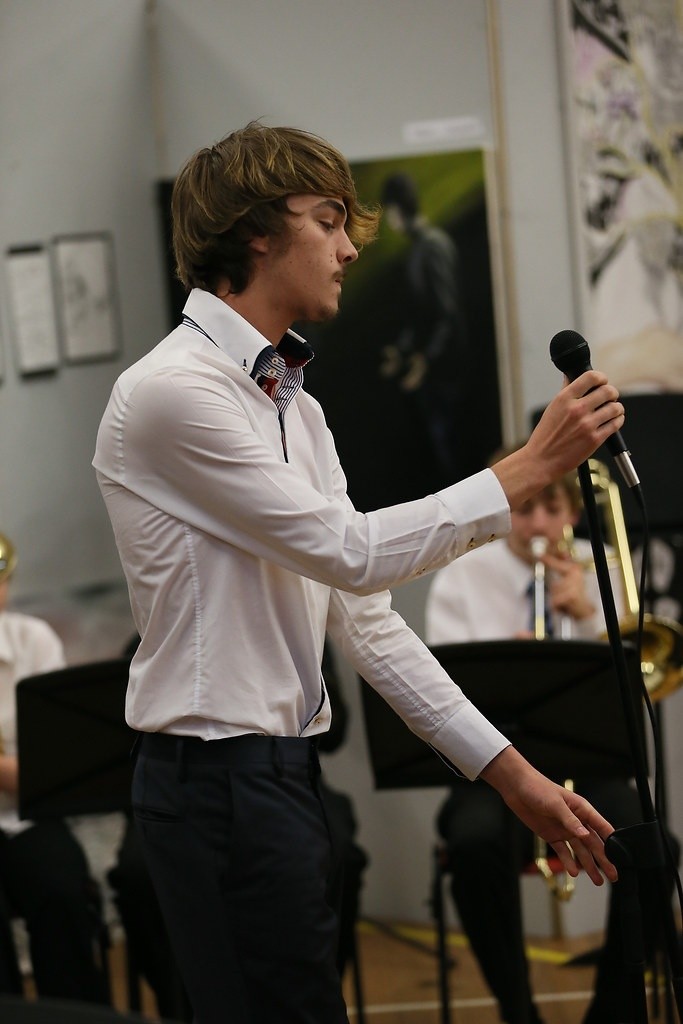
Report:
549,329,646,512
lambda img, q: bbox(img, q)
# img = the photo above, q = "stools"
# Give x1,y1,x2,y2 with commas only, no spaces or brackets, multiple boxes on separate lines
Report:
424,842,613,1024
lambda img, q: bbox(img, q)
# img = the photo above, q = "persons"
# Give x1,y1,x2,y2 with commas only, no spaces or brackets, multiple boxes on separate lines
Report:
424,446,678,1024
1,539,111,1024
92,119,625,1023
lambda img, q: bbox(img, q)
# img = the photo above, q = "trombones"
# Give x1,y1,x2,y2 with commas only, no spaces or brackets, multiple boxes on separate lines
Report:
525,454,683,904
